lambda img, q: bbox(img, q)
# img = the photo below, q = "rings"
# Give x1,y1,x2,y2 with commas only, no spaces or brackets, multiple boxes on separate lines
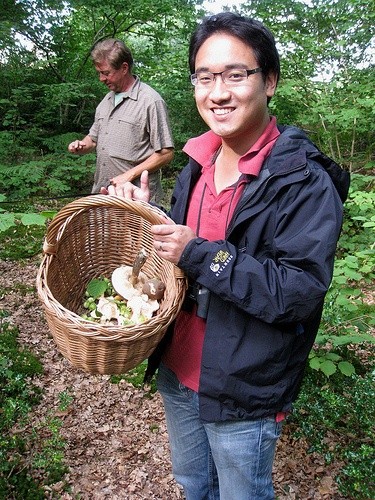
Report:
160,241,164,250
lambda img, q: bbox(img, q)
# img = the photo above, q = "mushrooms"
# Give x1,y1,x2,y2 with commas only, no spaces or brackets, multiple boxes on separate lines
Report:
111,248,166,302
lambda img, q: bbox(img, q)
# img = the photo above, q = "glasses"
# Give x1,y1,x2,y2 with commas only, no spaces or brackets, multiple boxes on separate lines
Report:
190,67,263,86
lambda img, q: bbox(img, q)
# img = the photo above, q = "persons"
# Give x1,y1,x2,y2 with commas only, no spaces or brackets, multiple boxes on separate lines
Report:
67,39,177,203
99,12,350,500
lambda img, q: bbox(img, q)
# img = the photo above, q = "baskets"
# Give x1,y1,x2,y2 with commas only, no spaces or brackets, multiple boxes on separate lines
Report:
35,192,189,374
